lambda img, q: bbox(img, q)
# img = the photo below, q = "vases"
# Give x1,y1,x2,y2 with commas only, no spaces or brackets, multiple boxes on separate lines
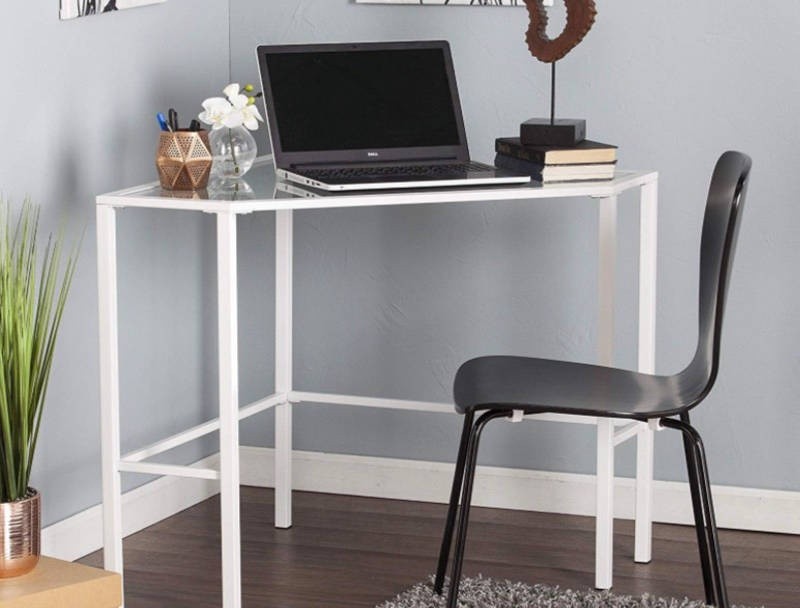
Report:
207,125,257,178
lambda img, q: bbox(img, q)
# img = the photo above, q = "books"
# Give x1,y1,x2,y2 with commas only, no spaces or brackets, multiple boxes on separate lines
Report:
494,137,618,183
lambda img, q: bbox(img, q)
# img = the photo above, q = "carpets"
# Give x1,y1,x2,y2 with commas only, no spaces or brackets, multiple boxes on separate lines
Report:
375,574,767,608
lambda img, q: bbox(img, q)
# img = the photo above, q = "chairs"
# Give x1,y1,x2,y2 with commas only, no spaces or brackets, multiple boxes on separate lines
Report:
434,151,752,608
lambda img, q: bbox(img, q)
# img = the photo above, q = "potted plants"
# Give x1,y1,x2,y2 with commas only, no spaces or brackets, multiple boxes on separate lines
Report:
0,193,85,581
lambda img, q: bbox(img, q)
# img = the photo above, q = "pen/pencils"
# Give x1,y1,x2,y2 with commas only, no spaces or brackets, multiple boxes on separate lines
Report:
157,112,167,131
191,120,200,131
164,121,172,132
169,108,178,131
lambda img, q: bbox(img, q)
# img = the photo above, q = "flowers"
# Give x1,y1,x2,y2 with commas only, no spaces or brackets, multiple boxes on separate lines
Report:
199,81,264,173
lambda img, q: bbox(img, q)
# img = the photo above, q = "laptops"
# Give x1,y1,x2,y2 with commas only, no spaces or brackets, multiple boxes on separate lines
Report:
256,40,531,191
273,179,332,198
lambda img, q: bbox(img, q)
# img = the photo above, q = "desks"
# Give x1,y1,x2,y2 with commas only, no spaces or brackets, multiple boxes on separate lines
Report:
94,152,660,608
